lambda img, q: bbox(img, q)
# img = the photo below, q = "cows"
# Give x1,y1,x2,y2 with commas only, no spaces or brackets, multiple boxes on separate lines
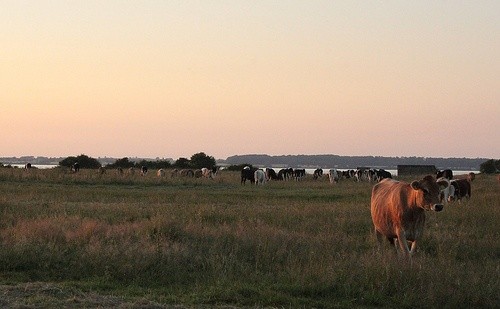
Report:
451,179,471,204
117,167,123,176
71,163,80,173
436,177,455,205
157,168,219,179
141,166,148,176
240,165,391,186
129,167,135,176
436,169,452,180
370,175,449,257
98,166,106,174
468,172,475,181
